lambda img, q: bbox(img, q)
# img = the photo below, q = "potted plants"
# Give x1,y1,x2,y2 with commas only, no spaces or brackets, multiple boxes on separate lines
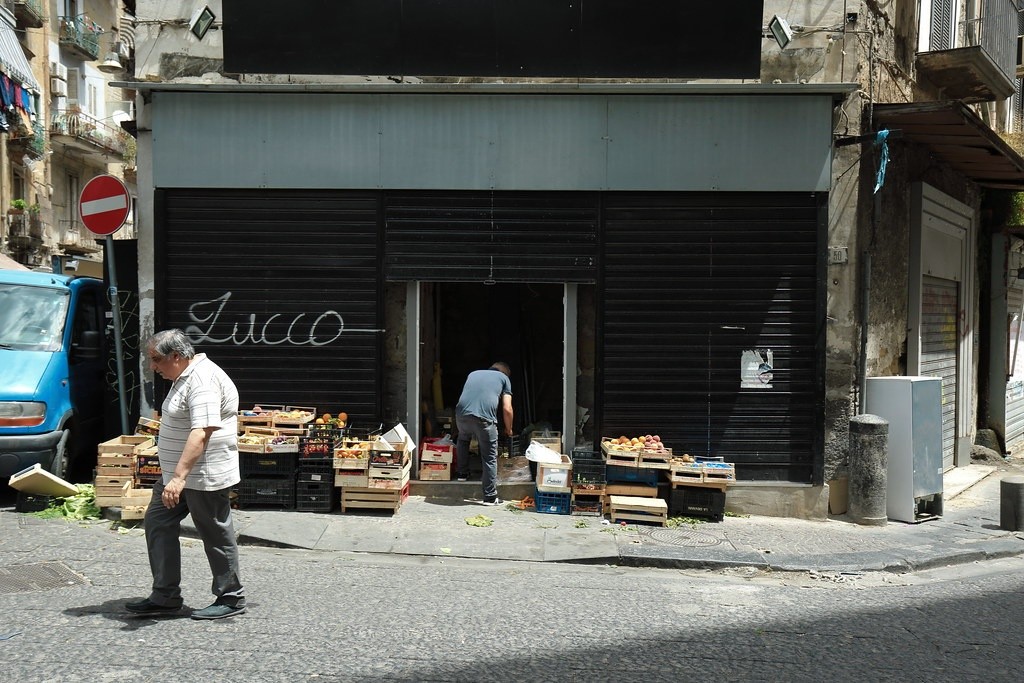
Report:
8,199,27,213
29,203,41,219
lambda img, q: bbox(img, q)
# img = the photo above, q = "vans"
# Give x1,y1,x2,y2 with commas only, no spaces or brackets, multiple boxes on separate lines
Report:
0,270,111,482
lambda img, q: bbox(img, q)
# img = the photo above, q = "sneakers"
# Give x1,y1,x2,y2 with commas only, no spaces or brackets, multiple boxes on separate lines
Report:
124,598,183,616
190,598,247,619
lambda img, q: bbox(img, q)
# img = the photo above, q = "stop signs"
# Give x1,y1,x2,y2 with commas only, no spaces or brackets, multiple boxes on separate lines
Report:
79,175,130,235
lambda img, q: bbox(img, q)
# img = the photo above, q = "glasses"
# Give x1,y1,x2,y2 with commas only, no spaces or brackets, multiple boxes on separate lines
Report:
148,351,169,364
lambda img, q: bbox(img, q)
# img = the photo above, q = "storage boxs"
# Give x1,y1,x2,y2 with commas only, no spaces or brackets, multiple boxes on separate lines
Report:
8,464,78,498
94,409,525,519
529,431,735,529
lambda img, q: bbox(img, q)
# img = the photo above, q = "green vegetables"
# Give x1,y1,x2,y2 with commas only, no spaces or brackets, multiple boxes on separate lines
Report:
33,483,104,521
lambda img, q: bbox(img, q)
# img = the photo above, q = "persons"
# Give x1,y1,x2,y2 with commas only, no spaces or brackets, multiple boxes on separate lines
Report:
124,329,247,618
456,361,514,505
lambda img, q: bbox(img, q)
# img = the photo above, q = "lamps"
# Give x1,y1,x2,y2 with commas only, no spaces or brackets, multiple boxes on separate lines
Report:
768,15,805,49
96,51,126,72
187,4,215,41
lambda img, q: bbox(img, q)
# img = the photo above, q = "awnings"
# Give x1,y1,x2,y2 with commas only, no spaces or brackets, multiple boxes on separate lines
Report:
120,17,135,49
107,80,862,191
0,17,41,96
866,100,1024,191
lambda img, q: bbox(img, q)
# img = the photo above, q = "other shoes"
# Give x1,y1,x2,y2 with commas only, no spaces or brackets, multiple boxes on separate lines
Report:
482,495,504,506
456,472,471,482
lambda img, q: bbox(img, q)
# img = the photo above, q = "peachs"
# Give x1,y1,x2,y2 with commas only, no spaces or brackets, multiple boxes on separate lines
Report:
315,413,346,429
604,433,664,453
336,442,368,459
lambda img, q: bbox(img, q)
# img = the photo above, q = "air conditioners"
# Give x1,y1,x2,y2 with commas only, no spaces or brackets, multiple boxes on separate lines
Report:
51,62,68,81
51,78,68,97
115,41,130,58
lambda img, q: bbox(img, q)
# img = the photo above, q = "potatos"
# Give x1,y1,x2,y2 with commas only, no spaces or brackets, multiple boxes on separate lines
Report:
670,454,695,462
